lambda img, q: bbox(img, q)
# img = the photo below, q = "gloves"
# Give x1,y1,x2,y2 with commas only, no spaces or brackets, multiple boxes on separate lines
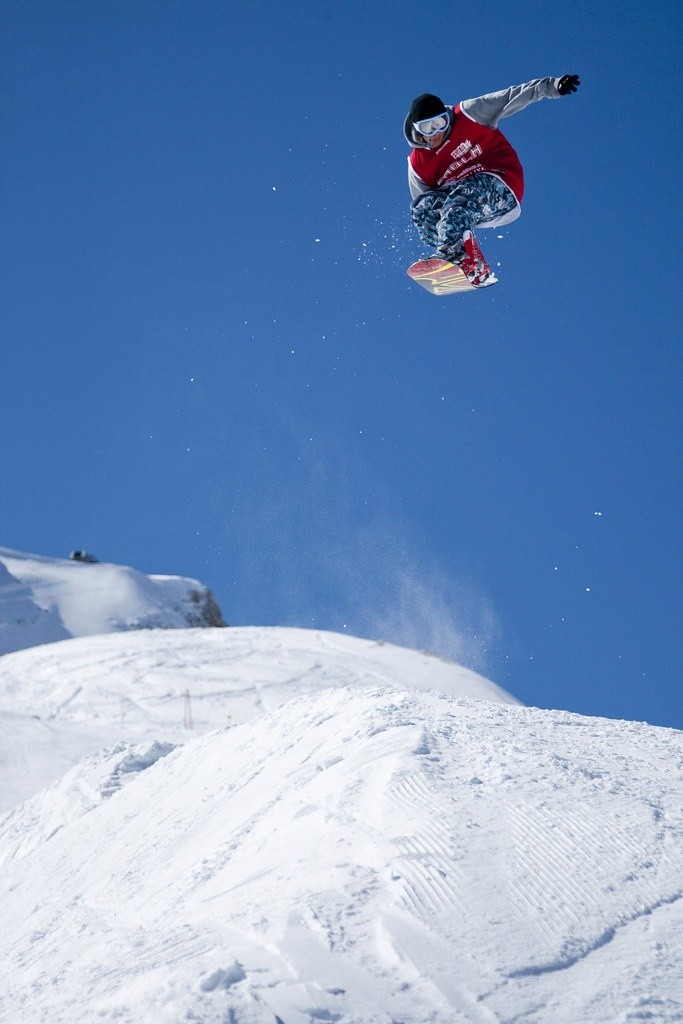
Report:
558,75,580,95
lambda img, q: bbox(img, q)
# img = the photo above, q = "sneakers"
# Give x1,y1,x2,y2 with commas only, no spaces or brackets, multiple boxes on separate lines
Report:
465,257,490,286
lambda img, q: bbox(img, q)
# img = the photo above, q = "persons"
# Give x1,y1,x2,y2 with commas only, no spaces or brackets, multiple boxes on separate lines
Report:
402,74,580,288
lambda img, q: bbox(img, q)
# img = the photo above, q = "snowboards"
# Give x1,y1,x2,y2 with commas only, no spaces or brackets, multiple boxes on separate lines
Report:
407,257,501,298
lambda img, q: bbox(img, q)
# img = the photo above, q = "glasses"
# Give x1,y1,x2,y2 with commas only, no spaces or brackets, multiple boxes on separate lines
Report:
415,113,450,135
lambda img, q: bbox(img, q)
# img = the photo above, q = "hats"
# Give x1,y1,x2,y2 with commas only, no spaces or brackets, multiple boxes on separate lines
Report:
410,94,446,123
